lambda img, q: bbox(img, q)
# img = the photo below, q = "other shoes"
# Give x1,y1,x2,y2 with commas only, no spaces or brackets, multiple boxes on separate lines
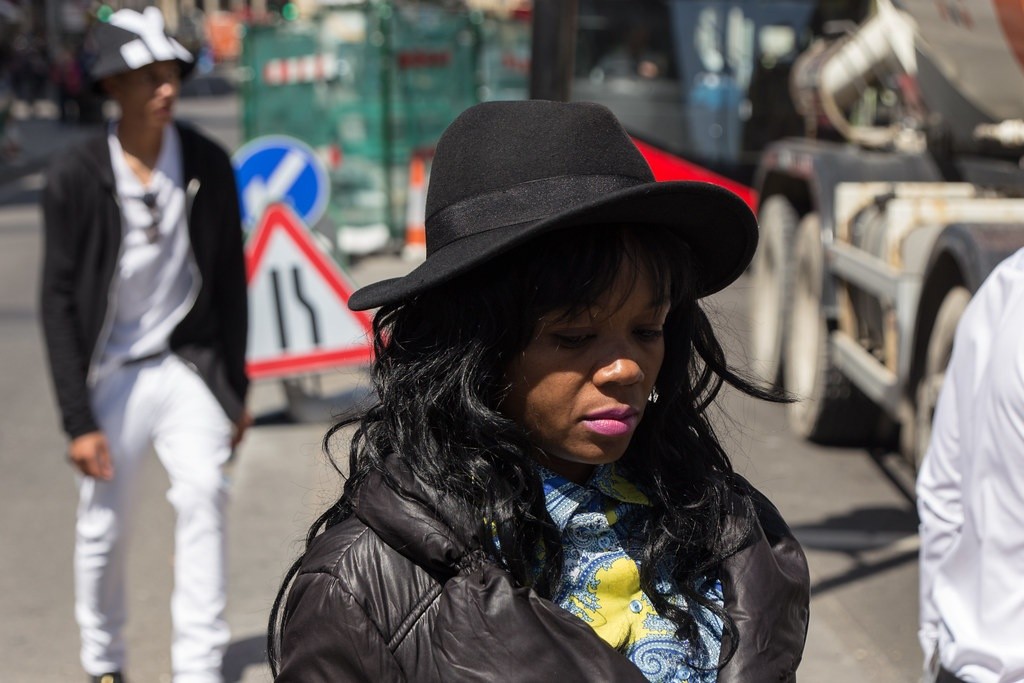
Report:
90,672,124,683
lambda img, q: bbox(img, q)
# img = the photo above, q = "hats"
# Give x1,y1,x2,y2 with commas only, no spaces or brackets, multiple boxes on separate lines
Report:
79,5,199,86
347,100,760,311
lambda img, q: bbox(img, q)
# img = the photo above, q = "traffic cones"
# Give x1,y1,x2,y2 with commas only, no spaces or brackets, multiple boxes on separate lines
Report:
399,157,436,266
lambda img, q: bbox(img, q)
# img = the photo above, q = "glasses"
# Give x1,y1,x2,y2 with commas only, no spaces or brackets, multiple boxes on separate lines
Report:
141,193,163,244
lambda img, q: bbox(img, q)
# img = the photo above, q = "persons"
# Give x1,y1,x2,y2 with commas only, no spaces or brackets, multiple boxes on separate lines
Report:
22,1,110,129
37,7,251,683
916,247,1024,683
267,101,810,683
